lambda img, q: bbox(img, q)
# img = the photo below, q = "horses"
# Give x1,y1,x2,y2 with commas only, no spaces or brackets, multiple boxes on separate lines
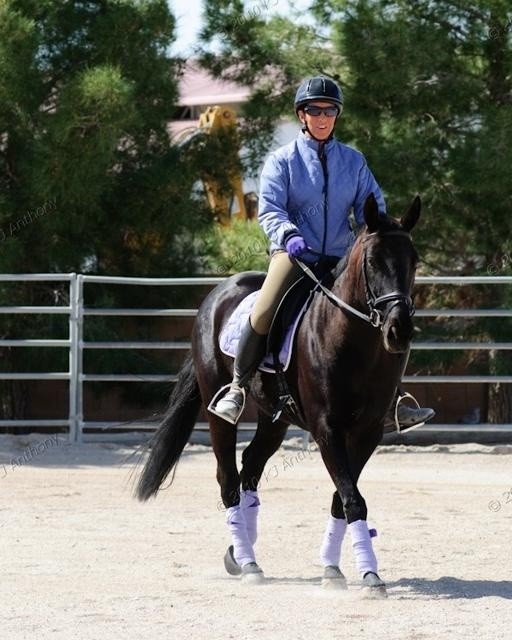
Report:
133,191,424,602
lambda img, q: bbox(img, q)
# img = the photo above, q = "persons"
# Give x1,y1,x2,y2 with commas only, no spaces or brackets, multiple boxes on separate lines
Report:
211,73,438,439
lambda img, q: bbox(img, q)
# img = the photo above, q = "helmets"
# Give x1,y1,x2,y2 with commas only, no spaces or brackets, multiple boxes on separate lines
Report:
295,76,344,118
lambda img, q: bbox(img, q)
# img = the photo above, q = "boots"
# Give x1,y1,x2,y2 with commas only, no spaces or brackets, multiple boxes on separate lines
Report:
214,314,271,419
382,393,435,434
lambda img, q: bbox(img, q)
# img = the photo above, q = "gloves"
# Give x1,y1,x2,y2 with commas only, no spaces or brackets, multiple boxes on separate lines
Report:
286,236,308,257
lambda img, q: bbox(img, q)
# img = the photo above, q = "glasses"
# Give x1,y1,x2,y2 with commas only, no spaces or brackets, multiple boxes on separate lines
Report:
303,105,339,117
